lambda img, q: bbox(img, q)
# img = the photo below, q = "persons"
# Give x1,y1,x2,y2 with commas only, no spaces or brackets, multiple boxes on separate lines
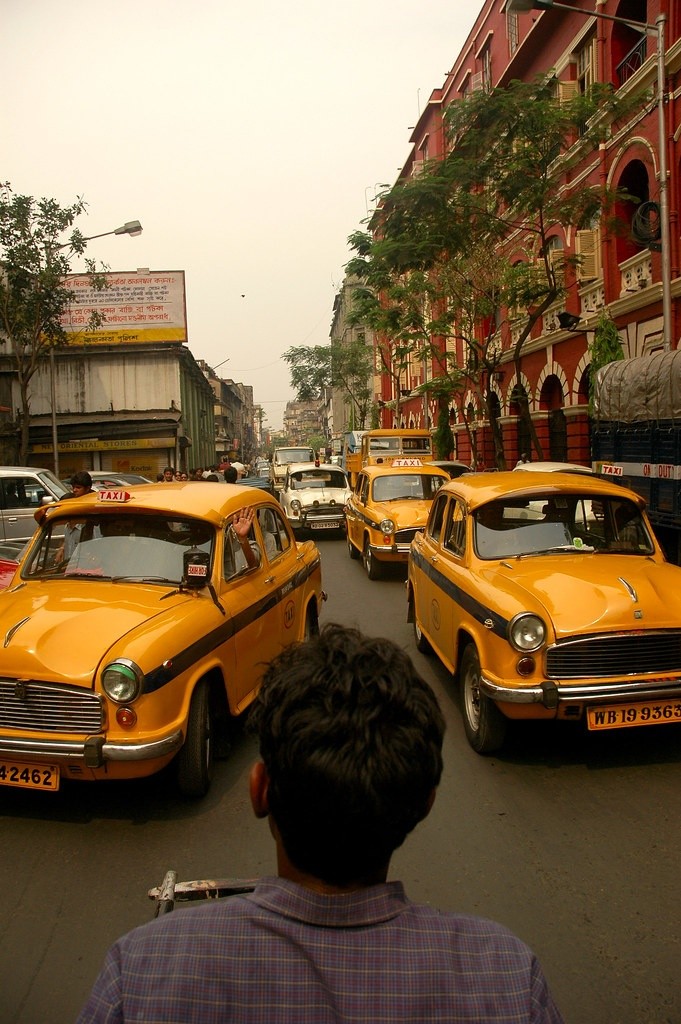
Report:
77,623,563,1024
54,452,530,575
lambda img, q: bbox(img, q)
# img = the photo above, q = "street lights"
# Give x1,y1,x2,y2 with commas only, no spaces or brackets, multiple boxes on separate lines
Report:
38,220,144,477
500,1,671,355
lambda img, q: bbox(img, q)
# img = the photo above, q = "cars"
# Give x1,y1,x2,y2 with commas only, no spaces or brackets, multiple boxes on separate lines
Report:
280,459,357,540
404,470,681,754
422,460,473,490
0,481,328,805
1,468,166,593
341,459,465,580
228,445,346,509
513,461,605,524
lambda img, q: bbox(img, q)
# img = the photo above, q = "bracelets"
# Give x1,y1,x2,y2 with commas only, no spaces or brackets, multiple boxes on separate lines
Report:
237,537,249,544
60,547,64,550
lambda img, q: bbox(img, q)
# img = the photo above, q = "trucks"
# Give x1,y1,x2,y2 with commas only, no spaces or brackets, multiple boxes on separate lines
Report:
340,427,435,492
589,352,681,540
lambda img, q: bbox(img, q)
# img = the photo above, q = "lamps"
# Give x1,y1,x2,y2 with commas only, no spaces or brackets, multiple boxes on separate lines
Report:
377,400,387,407
556,311,597,333
400,390,424,397
495,371,506,382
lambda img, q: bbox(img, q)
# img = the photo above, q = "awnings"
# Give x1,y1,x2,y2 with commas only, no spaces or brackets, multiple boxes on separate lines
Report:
15,412,183,439
